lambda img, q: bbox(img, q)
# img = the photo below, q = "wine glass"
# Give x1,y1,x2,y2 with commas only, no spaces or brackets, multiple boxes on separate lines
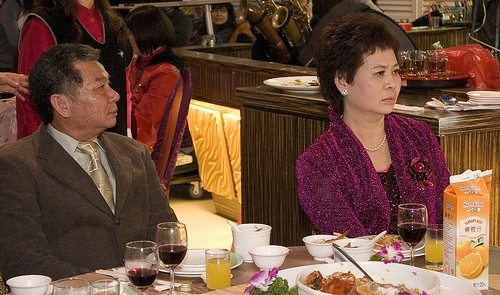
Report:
397,204,429,268
155,221,188,295
124,241,160,295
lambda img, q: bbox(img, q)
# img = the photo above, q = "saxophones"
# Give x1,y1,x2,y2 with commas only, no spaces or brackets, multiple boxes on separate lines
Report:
272,0,313,56
235,0,295,64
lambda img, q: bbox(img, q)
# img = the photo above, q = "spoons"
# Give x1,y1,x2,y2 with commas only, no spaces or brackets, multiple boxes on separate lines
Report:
332,242,395,288
227,220,243,233
355,230,387,248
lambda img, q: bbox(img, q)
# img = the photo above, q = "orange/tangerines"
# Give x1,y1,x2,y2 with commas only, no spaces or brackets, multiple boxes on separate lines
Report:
456,241,489,279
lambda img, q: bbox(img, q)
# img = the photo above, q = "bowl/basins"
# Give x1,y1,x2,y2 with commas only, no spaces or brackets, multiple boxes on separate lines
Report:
301,235,346,260
6,274,52,295
231,224,272,263
248,245,290,271
332,239,375,261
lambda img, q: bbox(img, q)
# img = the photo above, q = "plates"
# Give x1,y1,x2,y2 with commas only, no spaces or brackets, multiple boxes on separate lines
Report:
467,91,500,104
274,265,335,291
296,262,484,295
145,248,244,278
263,76,321,93
399,72,469,90
354,235,425,252
391,249,426,258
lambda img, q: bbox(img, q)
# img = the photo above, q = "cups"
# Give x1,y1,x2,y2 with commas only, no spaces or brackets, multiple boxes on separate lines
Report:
424,223,443,272
396,50,449,79
430,9,442,29
91,278,120,295
204,248,231,290
53,278,90,295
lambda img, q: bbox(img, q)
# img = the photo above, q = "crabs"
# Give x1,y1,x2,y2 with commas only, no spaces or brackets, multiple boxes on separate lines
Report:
304,270,385,295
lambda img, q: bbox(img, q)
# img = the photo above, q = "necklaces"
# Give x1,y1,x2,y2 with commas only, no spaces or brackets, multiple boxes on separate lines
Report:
340,112,388,151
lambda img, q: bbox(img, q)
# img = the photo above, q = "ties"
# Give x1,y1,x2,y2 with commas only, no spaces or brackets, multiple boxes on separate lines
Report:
78,140,116,215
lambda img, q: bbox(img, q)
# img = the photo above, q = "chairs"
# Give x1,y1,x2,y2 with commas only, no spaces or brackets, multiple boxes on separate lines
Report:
145,67,193,199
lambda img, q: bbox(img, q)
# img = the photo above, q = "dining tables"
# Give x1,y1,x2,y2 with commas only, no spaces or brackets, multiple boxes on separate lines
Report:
2,233,499,294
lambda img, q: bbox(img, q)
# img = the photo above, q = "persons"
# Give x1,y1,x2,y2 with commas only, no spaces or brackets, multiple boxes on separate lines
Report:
0,43,182,290
0,71,33,102
190,1,237,46
15,0,135,140
150,0,193,48
0,0,27,150
125,4,185,158
296,11,452,240
